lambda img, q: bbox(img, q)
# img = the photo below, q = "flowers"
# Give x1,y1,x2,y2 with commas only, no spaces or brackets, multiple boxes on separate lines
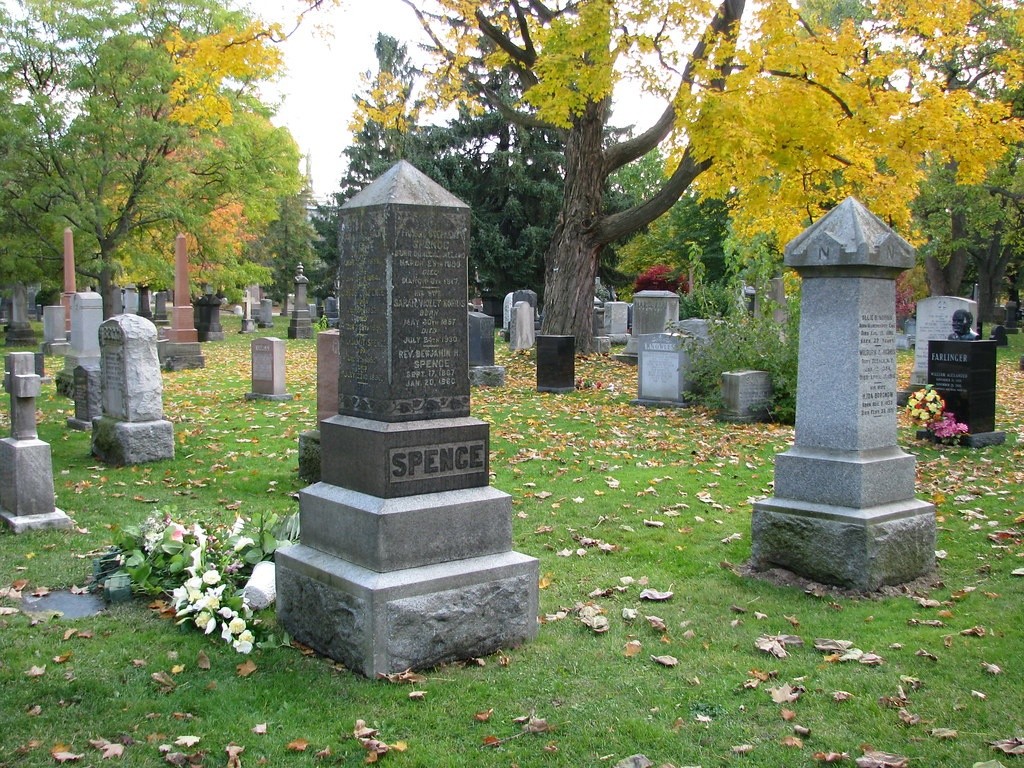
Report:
86,508,299,654
905,385,968,446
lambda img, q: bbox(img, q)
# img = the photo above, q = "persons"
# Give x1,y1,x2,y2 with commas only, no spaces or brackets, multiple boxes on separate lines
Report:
948,309,980,340
605,286,618,301
469,294,482,312
215,290,224,299
